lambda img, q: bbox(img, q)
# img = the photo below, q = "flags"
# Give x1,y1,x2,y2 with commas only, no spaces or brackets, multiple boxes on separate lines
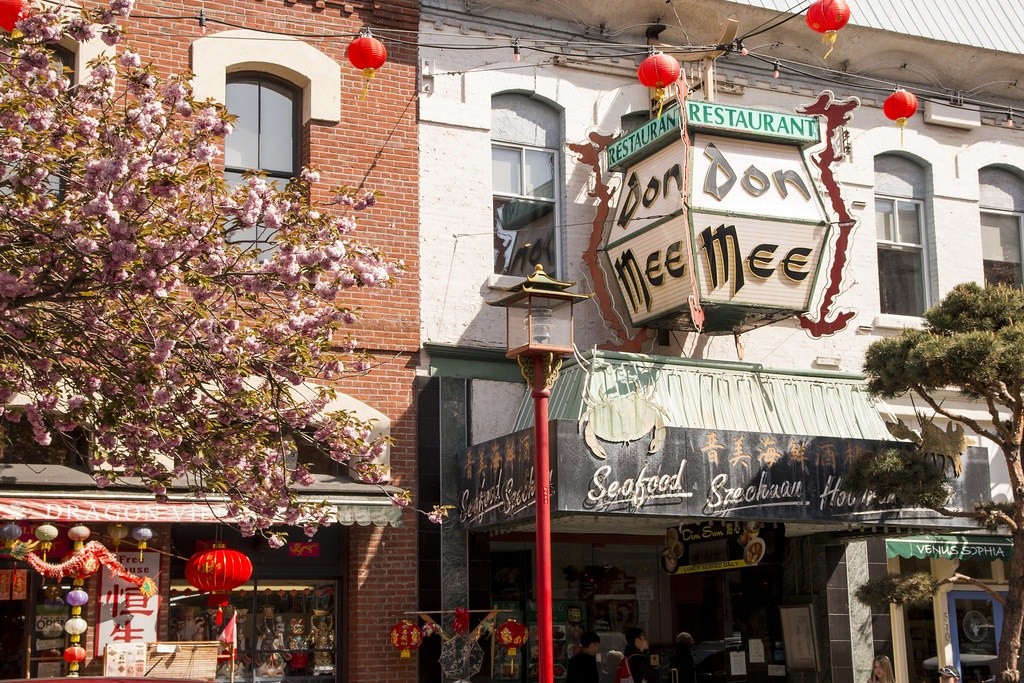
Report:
217,612,239,660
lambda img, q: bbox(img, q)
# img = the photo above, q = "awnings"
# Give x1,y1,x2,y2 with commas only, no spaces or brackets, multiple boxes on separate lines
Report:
886,536,1015,563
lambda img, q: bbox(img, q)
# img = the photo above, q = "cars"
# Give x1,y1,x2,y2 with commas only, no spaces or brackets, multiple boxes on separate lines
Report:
690,633,787,675
922,653,1001,683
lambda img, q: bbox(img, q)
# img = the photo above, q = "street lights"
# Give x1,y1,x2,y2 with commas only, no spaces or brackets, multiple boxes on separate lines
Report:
486,262,594,682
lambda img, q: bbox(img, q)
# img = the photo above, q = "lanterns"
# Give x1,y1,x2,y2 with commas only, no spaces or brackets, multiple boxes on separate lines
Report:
806,0,850,59
882,89,919,145
1,520,152,671
346,34,387,101
387,619,423,658
0,1,33,40
184,543,253,626
494,618,529,674
637,51,681,120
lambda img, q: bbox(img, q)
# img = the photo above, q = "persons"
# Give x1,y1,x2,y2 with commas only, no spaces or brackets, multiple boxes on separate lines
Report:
938,665,960,683
867,655,896,683
669,632,696,683
622,627,661,683
566,631,601,683
